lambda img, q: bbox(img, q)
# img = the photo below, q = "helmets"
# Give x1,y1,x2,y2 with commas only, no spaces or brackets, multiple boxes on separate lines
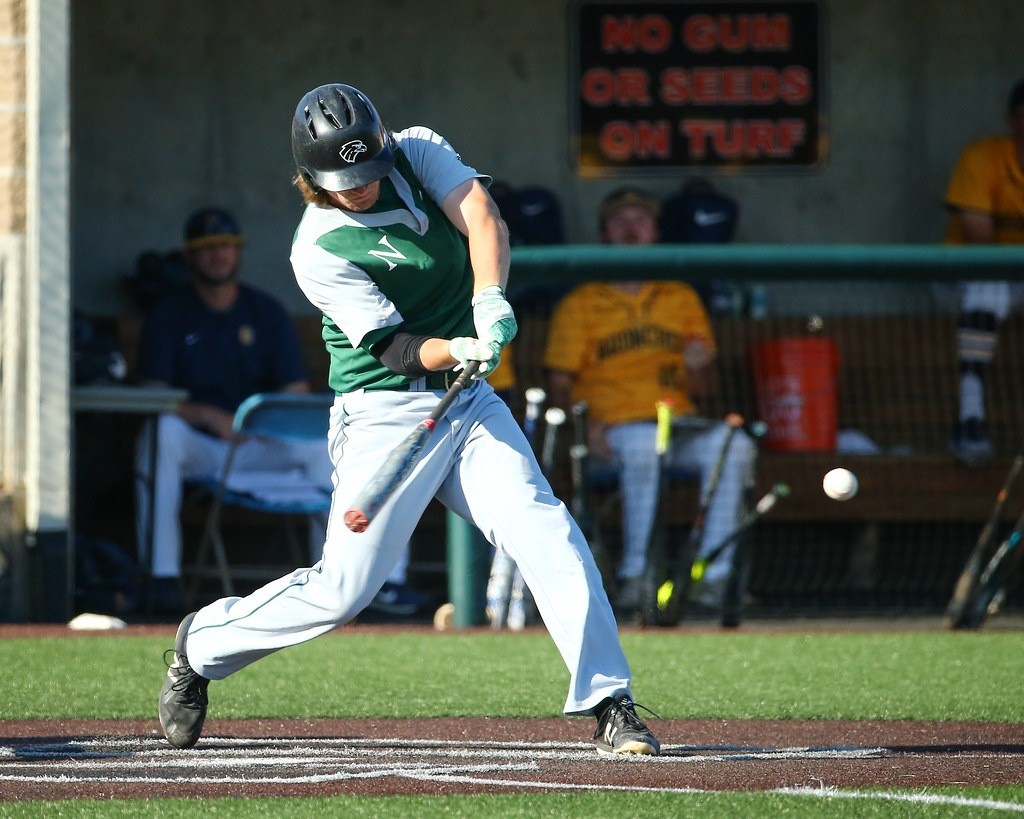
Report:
291,85,395,193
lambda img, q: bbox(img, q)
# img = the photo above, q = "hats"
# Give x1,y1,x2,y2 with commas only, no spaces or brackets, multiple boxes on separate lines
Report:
597,189,664,228
185,211,243,256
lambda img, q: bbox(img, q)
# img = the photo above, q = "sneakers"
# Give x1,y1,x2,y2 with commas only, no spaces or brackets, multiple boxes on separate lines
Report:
593,697,658,753
159,611,207,749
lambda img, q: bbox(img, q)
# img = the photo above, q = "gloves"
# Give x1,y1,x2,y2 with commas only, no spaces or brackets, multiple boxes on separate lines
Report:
448,335,501,380
473,289,519,349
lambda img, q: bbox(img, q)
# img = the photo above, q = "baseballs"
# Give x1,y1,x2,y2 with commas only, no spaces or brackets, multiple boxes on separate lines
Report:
823,468,859,500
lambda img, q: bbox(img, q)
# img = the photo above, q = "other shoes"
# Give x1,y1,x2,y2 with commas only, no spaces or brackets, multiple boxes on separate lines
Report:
370,584,429,619
617,580,642,608
692,582,752,615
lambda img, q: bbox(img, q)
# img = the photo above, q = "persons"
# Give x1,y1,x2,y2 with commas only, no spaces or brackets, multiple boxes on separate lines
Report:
158,83,661,756
134,207,428,615
542,184,758,630
943,79,1024,453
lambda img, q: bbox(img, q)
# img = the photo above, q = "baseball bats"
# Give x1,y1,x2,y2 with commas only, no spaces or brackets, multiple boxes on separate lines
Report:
946,455,1024,629
506,407,567,630
660,413,745,626
639,401,670,627
570,401,591,545
486,388,546,621
656,482,791,612
343,360,480,533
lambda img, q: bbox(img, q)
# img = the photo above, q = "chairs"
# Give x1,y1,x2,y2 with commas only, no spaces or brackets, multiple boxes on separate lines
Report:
183,393,330,604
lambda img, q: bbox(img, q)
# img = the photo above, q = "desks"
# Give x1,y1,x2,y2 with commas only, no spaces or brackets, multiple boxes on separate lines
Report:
67,384,187,571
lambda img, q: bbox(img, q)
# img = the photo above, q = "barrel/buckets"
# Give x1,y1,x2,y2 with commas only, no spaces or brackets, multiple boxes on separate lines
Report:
748,333,839,454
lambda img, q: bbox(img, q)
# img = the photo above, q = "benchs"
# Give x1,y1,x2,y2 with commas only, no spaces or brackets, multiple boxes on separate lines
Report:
513,291,1024,620
